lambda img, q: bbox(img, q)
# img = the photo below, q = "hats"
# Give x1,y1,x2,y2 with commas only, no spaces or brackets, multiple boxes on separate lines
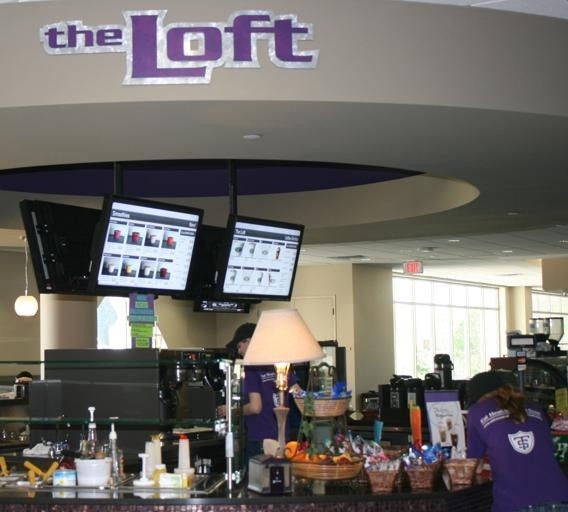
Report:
464,372,514,409
226,324,256,349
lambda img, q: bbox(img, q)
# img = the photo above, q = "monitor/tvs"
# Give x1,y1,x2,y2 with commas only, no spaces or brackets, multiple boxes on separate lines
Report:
17,194,304,313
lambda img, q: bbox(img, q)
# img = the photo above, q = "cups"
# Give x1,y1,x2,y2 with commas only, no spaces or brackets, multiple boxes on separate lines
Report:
227,241,281,287
437,417,459,448
108,230,173,278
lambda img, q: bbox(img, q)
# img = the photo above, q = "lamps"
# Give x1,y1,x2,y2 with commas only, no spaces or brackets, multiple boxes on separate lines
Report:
12,234,38,317
240,308,326,463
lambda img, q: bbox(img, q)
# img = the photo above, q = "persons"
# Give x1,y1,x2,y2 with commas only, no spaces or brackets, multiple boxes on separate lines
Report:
215,322,278,488
460,370,568,512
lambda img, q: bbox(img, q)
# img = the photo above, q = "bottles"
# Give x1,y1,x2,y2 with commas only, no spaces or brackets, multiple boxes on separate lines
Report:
178,434,190,469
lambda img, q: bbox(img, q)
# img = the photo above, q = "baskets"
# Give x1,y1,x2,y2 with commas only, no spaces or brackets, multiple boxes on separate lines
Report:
407,462,441,490
445,458,484,486
294,398,350,417
290,462,364,479
366,470,405,494
